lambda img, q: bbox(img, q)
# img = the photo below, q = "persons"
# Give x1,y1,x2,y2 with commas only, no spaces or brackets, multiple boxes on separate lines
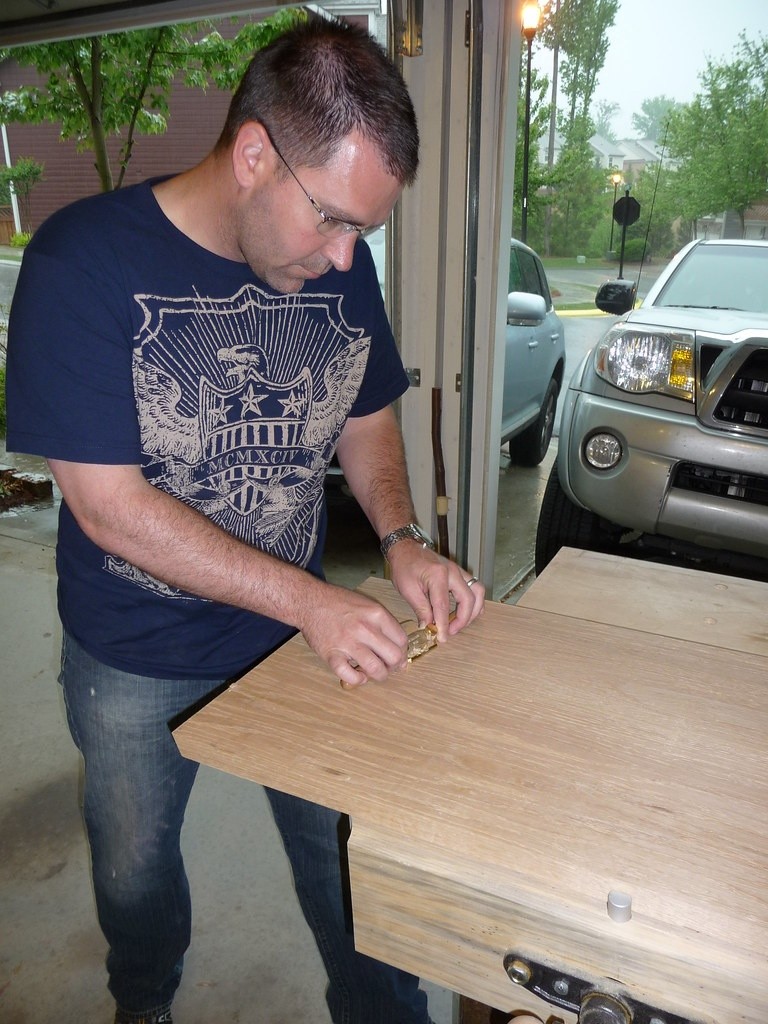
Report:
4,17,486,1024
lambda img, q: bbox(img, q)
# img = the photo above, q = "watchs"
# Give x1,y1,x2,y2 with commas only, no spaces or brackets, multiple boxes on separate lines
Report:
379,523,436,561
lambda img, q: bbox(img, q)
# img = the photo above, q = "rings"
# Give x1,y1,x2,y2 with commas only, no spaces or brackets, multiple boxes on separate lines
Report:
466,577,480,587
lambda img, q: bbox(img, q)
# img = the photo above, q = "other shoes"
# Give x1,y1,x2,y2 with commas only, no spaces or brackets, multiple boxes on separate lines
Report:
114,1005,173,1024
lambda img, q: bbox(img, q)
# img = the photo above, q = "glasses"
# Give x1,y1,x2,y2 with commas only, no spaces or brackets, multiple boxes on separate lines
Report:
269,137,386,240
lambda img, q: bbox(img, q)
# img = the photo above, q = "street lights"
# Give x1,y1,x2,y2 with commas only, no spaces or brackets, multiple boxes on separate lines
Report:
522,2,542,243
609,172,624,255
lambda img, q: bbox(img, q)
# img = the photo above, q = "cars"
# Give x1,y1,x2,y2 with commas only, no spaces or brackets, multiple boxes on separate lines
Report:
323,227,565,475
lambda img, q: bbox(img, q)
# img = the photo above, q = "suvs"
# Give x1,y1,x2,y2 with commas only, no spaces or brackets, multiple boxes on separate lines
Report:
536,236,768,577
